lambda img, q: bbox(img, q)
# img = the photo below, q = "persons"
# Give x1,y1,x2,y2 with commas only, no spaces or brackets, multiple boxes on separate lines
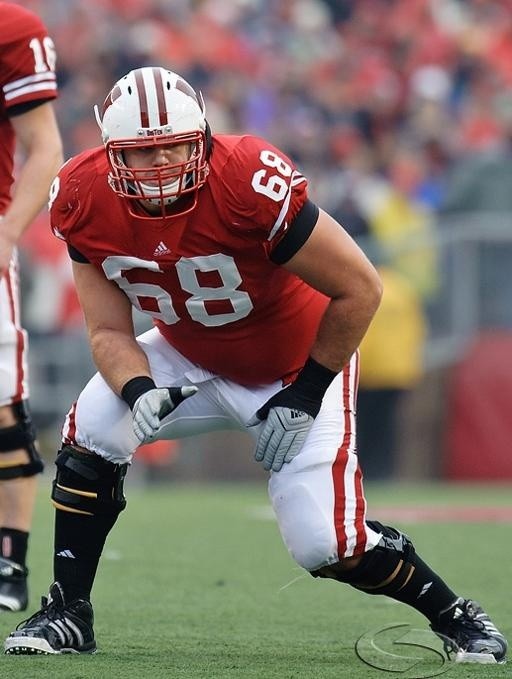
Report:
2,66,510,668
0,0,66,614
0,1,511,482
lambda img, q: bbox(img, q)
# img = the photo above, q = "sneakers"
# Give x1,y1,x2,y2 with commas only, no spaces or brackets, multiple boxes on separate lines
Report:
4,581,97,656
0,556,29,612
427,596,508,664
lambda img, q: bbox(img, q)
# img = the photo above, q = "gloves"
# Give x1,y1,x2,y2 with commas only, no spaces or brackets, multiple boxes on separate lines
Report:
245,353,339,472
122,376,198,443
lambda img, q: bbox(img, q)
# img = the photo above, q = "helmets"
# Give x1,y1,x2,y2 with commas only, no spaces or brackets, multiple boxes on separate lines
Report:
94,67,211,222
